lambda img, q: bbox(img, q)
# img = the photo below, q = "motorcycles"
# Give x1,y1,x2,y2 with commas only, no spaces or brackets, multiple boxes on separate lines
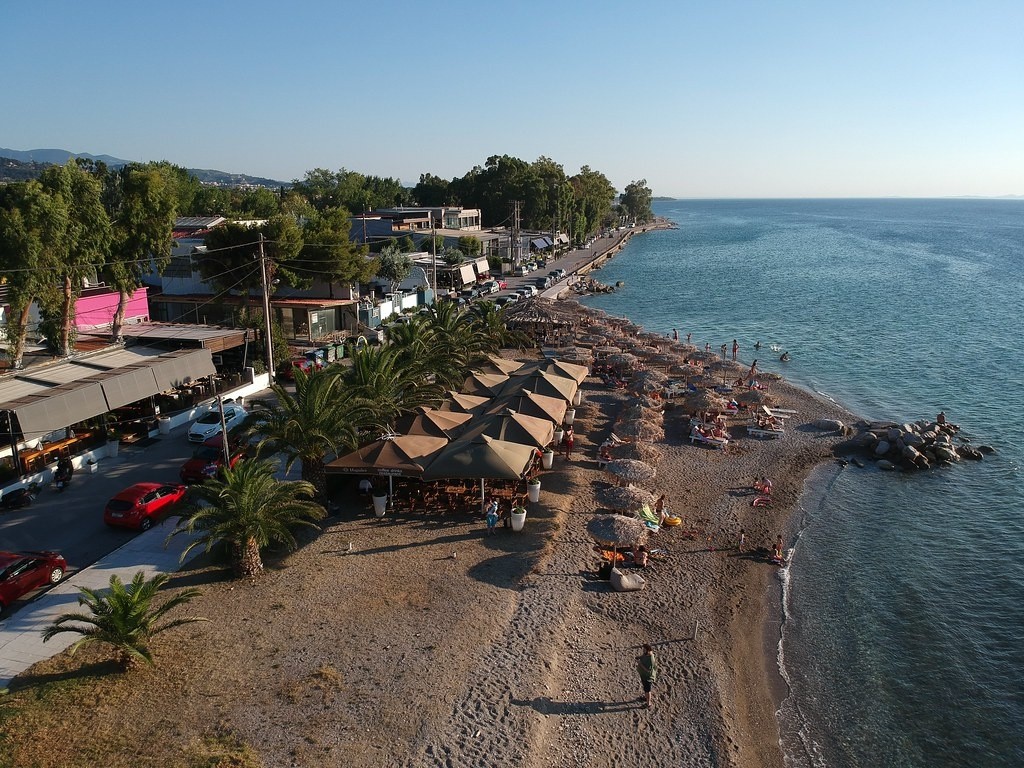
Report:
55,458,73,492
0,488,35,511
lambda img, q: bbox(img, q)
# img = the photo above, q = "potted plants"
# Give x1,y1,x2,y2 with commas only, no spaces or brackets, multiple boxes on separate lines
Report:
566,407,576,425
528,478,541,503
371,489,387,518
554,428,564,445
511,506,527,531
542,448,554,470
574,389,582,406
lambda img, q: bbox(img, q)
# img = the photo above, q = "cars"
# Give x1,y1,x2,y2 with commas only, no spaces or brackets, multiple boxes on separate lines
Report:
178,432,252,483
375,223,637,331
276,355,324,382
104,480,189,531
188,403,249,444
0,549,66,617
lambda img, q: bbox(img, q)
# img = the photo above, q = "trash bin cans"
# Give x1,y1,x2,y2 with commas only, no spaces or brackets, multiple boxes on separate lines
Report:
305,349,324,372
320,346,336,363
333,343,344,360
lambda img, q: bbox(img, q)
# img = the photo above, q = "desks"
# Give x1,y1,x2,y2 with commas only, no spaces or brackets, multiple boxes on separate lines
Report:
445,485,513,503
20,432,94,473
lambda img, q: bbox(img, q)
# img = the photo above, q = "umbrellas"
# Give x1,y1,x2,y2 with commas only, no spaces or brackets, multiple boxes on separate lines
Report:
564,299,782,411
588,395,666,547
322,359,586,481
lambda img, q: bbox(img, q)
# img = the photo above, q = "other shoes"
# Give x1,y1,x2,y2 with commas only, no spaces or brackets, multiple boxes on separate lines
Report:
640,703,652,711
636,696,647,702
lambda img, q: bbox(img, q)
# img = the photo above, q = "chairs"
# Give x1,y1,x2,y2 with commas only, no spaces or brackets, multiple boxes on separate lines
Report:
595,375,797,592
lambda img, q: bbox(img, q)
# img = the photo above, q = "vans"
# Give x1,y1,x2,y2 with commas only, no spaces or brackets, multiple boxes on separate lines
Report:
345,335,368,355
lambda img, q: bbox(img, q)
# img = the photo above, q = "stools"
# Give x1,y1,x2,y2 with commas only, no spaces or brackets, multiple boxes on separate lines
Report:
62,445,70,456
73,440,82,452
43,451,51,465
28,458,36,472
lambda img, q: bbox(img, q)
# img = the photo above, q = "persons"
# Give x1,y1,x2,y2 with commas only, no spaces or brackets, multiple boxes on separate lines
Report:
486,497,497,536
687,332,692,339
936,411,946,423
721,343,727,353
732,339,739,356
655,495,665,527
780,352,789,361
566,424,574,461
697,413,729,443
757,415,784,432
738,529,746,551
771,535,785,561
672,328,678,339
705,343,711,352
635,546,648,566
751,359,758,374
754,478,771,492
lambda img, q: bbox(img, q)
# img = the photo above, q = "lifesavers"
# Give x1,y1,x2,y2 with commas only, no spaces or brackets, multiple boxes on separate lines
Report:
665,516,681,526
